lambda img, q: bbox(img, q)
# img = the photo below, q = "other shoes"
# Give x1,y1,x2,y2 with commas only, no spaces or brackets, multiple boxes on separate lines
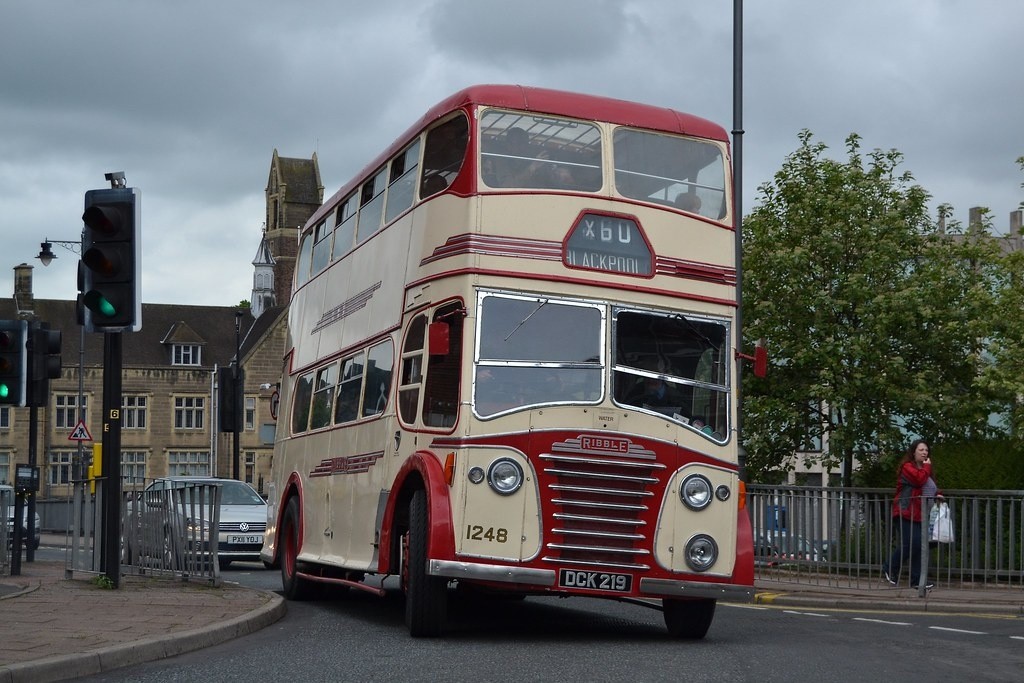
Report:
885,569,898,584
912,581,933,588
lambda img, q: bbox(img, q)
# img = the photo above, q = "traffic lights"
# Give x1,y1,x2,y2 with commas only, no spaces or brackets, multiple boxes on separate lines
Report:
26,322,64,407
0,318,27,408
82,186,142,333
81,450,94,481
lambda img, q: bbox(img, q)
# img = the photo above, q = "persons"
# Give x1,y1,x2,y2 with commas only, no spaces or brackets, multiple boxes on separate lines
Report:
675,193,702,215
427,329,569,427
495,127,575,189
623,356,691,418
884,439,945,589
423,175,447,195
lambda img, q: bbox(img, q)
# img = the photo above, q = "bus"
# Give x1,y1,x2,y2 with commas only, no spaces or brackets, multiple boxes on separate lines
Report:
255,82,768,637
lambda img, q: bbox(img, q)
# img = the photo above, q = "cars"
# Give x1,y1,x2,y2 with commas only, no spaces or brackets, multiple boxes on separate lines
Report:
0,485,40,550
116,473,270,572
753,528,829,563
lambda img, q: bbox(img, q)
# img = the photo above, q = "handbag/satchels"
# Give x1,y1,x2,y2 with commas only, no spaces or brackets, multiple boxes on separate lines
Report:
928,502,954,543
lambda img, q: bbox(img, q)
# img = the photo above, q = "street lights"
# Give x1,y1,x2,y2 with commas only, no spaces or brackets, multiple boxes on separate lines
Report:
34,239,84,479
